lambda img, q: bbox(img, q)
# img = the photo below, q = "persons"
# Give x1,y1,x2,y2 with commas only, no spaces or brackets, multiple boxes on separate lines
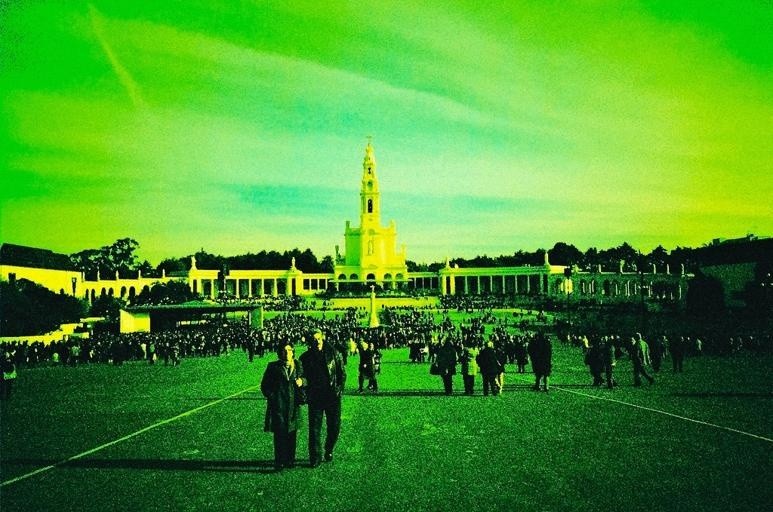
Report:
0,290,772,393
259,340,297,470
295,325,344,466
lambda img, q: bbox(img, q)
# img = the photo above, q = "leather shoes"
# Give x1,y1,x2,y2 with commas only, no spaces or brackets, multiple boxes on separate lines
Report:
275,451,333,469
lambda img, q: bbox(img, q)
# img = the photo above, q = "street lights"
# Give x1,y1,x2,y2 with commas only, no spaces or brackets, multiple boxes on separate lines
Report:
72,278,77,297
564,268,572,324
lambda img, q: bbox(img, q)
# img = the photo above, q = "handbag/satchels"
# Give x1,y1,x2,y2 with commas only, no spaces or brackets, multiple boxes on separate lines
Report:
292,358,307,407
